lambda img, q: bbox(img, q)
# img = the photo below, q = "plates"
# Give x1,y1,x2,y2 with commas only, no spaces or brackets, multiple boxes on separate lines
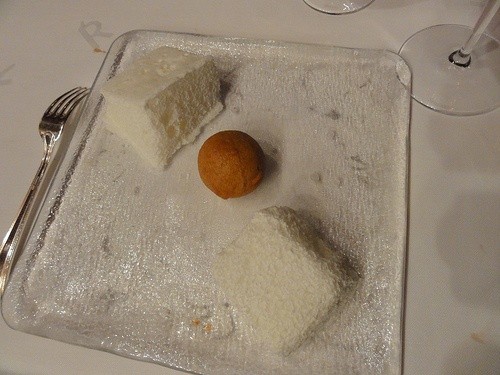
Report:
1,26,412,374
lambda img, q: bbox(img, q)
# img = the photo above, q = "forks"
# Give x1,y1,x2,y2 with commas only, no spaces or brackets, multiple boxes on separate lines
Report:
1,85,90,298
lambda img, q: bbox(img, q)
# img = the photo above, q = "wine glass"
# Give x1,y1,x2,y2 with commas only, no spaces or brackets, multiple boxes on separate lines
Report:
394,0,500,117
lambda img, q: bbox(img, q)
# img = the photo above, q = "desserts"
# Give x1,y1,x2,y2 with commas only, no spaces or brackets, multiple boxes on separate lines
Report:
97,46,227,173
197,128,266,201
210,205,359,359
